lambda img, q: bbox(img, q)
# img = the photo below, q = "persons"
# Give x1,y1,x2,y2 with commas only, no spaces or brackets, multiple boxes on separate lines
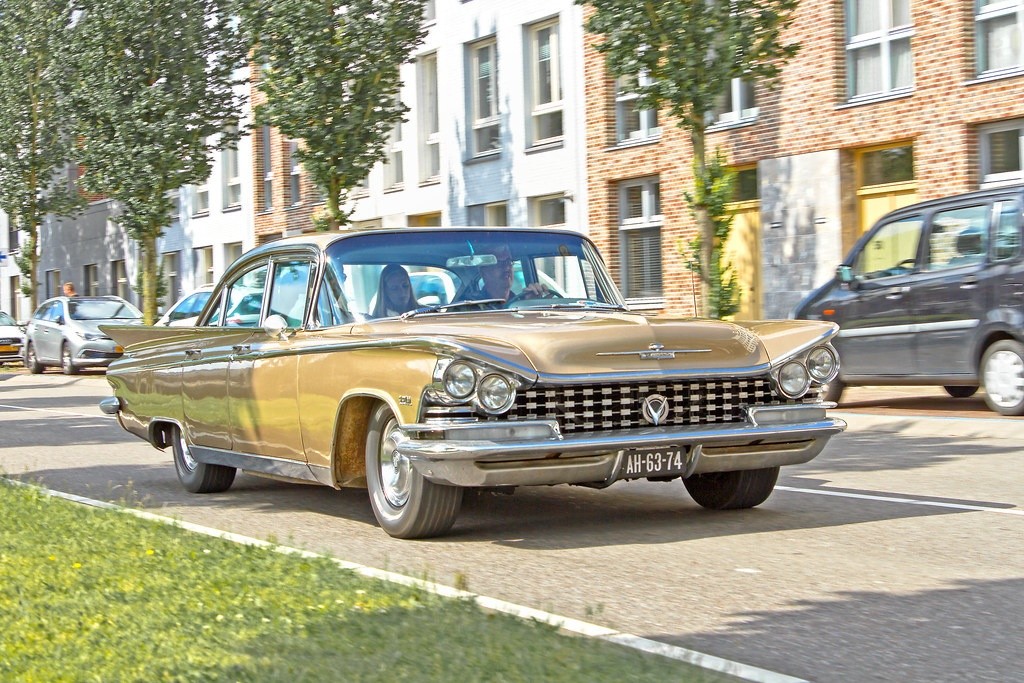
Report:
271,260,373,329
372,264,433,319
454,244,555,314
63,282,80,296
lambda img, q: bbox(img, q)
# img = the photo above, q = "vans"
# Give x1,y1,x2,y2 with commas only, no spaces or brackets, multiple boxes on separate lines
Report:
790,184,1024,422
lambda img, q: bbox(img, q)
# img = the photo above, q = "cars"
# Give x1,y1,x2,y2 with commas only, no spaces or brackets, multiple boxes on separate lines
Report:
22,295,147,375
95,222,850,541
0,311,27,365
150,282,264,328
